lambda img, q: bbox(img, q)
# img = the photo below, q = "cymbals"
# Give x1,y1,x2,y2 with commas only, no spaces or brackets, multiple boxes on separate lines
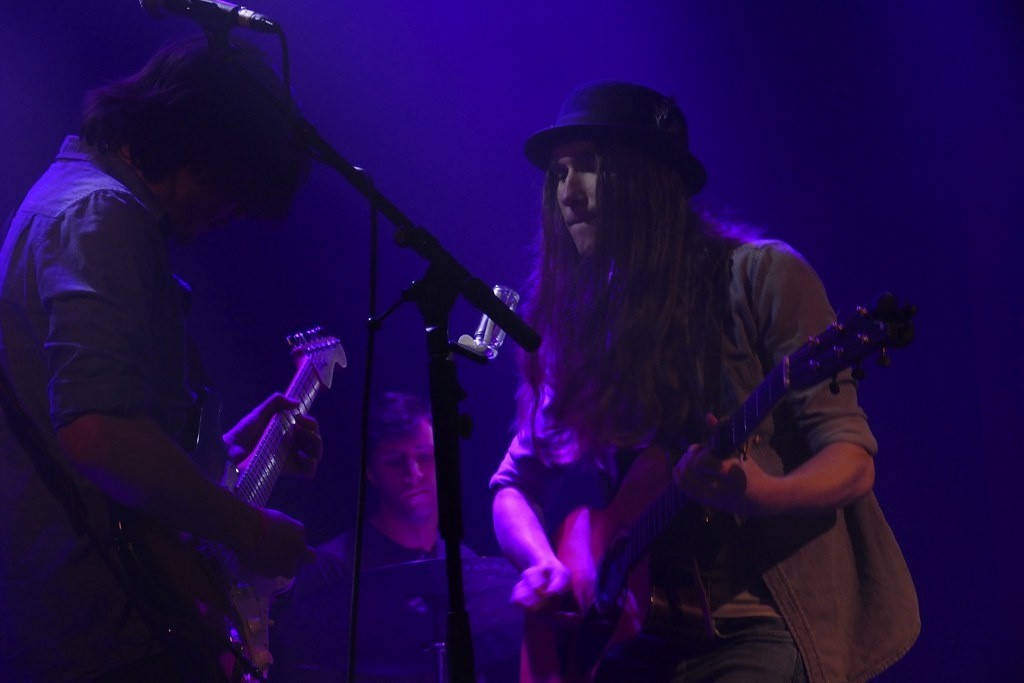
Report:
276,555,530,662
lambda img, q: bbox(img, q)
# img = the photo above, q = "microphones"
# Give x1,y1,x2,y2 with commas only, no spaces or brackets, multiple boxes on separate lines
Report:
140,0,274,32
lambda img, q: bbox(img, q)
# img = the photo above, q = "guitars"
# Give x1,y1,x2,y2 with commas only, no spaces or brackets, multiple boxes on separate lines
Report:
513,294,919,680
109,313,351,682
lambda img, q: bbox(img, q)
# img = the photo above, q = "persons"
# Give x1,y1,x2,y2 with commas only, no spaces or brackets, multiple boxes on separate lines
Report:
0,39,323,683
490,85,921,683
269,392,524,683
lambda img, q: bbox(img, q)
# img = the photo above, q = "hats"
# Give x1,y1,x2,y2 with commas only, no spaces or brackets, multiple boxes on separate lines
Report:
525,81,707,185
